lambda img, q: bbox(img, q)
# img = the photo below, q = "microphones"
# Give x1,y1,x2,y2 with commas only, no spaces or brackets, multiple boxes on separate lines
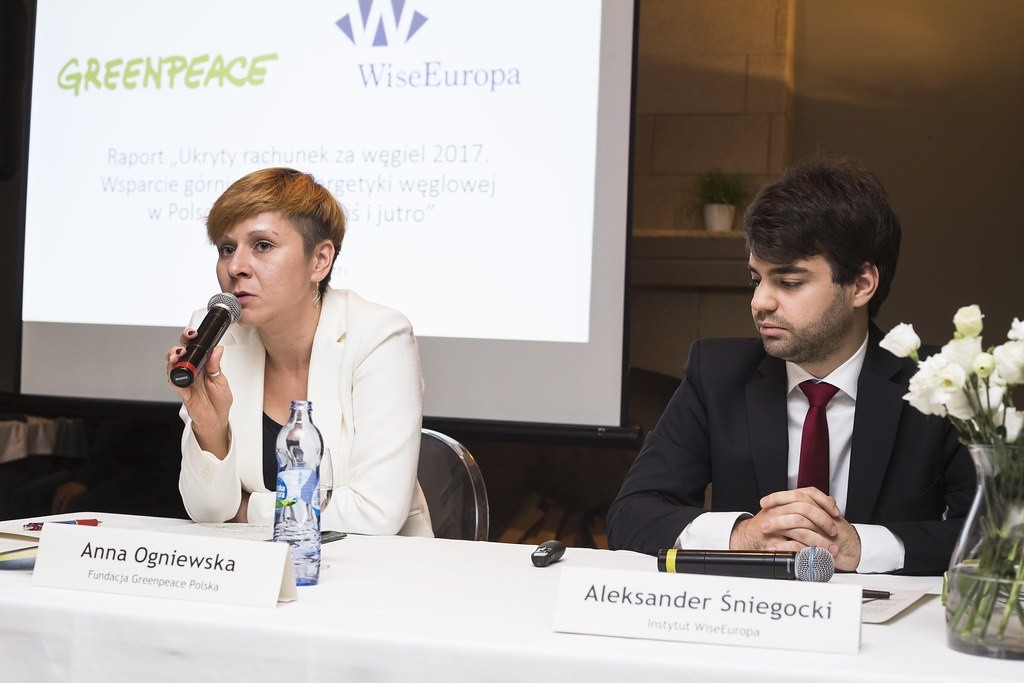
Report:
657,545,836,584
169,293,242,388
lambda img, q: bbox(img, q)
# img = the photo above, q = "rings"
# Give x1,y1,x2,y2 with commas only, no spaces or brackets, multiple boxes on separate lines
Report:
206,369,222,377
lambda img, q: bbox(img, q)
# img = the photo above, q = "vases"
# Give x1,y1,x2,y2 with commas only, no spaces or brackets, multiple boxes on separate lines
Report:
943,445,1024,660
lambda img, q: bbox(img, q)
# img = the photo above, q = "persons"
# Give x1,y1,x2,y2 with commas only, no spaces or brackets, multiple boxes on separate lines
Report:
165,167,439,537
602,161,980,576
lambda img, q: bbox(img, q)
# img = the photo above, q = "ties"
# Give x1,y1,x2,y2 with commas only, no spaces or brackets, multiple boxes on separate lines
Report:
797,381,841,496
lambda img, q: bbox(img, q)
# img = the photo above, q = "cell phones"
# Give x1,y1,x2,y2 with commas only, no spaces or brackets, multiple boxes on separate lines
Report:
320,531,348,545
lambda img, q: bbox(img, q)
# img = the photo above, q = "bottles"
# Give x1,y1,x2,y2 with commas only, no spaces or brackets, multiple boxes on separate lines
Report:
275,400,323,587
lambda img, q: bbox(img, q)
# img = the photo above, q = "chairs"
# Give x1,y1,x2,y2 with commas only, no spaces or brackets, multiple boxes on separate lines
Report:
417,428,489,542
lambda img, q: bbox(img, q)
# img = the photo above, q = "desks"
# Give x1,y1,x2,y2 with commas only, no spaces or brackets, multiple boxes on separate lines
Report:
0,511,1024,683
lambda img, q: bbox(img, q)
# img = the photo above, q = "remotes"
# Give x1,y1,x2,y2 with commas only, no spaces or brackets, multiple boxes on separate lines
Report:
531,539,566,568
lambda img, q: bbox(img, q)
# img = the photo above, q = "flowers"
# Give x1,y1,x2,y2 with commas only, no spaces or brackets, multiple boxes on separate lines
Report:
879,304,1024,642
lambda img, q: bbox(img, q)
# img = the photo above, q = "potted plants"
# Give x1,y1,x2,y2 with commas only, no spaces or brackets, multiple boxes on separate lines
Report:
676,166,753,231
